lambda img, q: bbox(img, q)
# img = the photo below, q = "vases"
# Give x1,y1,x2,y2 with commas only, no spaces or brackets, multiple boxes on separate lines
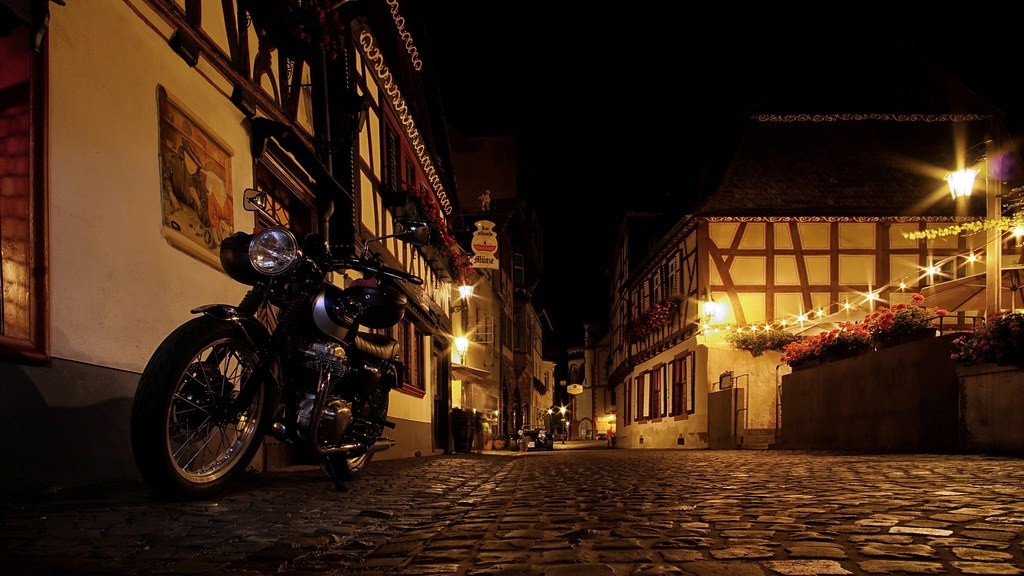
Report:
954,364,1024,454
790,327,936,372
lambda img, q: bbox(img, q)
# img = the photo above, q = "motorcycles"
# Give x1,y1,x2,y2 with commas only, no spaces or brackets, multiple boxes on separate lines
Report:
128,188,430,498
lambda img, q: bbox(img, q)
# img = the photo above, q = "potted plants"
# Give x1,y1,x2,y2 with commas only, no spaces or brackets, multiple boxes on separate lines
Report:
491,434,506,450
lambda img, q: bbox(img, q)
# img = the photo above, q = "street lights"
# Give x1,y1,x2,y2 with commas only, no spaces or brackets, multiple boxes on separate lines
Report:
560,405,567,444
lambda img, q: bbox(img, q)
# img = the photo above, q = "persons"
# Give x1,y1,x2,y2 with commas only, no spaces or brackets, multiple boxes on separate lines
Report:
607,429,613,446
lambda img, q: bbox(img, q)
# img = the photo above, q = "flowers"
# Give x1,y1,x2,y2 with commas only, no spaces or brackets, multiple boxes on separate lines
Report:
778,292,946,367
949,309,1024,371
